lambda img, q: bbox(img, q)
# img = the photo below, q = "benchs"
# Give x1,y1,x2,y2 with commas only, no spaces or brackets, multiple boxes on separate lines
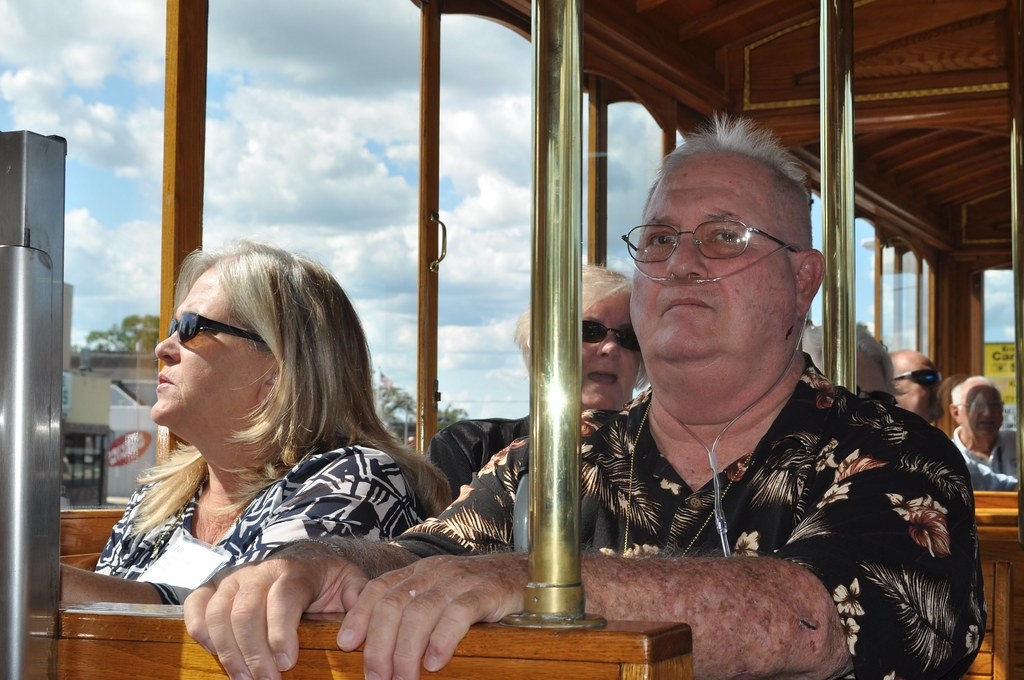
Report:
24,489,1024,680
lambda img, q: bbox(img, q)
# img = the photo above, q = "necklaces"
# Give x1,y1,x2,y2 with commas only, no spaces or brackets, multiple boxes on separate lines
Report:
623,401,753,559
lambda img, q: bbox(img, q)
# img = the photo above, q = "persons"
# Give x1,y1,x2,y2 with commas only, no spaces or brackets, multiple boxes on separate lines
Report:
422,267,649,504
58,242,454,605
183,126,987,680
805,321,1018,491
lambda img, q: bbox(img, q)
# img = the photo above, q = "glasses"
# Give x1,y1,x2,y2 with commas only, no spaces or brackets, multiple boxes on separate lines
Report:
582,319,642,352
954,400,1005,413
167,313,266,344
894,368,943,389
621,219,797,282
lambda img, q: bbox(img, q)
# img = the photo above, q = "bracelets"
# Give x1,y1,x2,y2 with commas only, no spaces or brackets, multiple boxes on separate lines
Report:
265,537,353,564
